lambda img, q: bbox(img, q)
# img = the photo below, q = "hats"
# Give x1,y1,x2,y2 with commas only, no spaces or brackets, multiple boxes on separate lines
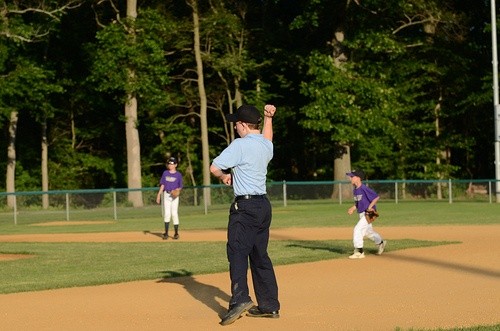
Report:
223,104,262,124
346,169,364,178
167,157,177,165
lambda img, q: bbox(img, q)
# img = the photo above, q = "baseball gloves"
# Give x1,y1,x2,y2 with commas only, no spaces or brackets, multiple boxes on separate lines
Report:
365,209,379,224
169,189,180,200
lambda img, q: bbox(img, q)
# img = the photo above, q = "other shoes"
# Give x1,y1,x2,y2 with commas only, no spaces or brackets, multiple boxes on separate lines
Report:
162,233,169,240
376,240,387,255
173,234,179,239
349,251,365,259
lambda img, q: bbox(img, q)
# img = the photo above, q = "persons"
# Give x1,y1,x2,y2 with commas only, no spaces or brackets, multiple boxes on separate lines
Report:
346,170,387,259
210,104,281,325
156,157,183,240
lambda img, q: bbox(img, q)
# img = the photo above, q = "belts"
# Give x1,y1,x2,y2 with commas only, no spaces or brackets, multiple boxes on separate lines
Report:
234,195,266,201
162,189,171,193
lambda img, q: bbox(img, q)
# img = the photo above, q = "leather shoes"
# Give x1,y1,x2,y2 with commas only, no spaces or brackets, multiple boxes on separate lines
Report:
247,305,279,319
222,298,254,325
